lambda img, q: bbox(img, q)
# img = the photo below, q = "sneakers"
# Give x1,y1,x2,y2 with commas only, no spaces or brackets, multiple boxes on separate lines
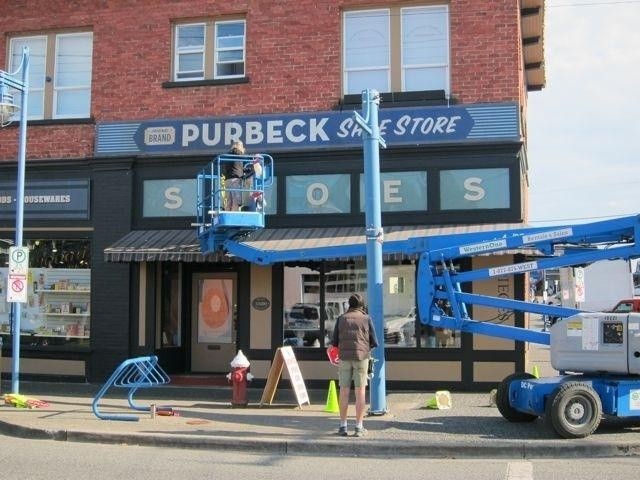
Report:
339,423,348,435
354,426,367,436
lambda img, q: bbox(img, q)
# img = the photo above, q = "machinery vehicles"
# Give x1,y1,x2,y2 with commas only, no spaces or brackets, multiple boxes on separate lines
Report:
188,148,640,441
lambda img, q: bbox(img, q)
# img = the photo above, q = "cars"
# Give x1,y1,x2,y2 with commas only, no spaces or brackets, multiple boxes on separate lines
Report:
634,282,640,298
543,285,561,329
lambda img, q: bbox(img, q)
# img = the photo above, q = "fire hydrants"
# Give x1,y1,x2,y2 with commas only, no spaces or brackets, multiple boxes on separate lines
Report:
223,347,255,409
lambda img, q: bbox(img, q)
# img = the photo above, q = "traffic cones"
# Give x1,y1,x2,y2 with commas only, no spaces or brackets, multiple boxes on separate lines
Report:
530,364,539,378
423,385,455,411
318,376,340,414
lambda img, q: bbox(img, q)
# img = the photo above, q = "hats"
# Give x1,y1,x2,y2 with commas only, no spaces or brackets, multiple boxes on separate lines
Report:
231,141,245,153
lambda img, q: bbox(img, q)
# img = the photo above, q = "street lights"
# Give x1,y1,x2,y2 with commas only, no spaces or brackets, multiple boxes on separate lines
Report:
0,45,36,395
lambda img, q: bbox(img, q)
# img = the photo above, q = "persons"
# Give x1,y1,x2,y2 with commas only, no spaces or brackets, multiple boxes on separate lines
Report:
434,327,447,348
241,191,268,213
331,293,379,438
225,141,259,212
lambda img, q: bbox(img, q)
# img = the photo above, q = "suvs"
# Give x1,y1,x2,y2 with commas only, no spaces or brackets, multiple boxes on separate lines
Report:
280,294,418,347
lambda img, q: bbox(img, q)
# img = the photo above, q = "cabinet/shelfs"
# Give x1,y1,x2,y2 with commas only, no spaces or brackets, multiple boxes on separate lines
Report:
33,280,90,339
